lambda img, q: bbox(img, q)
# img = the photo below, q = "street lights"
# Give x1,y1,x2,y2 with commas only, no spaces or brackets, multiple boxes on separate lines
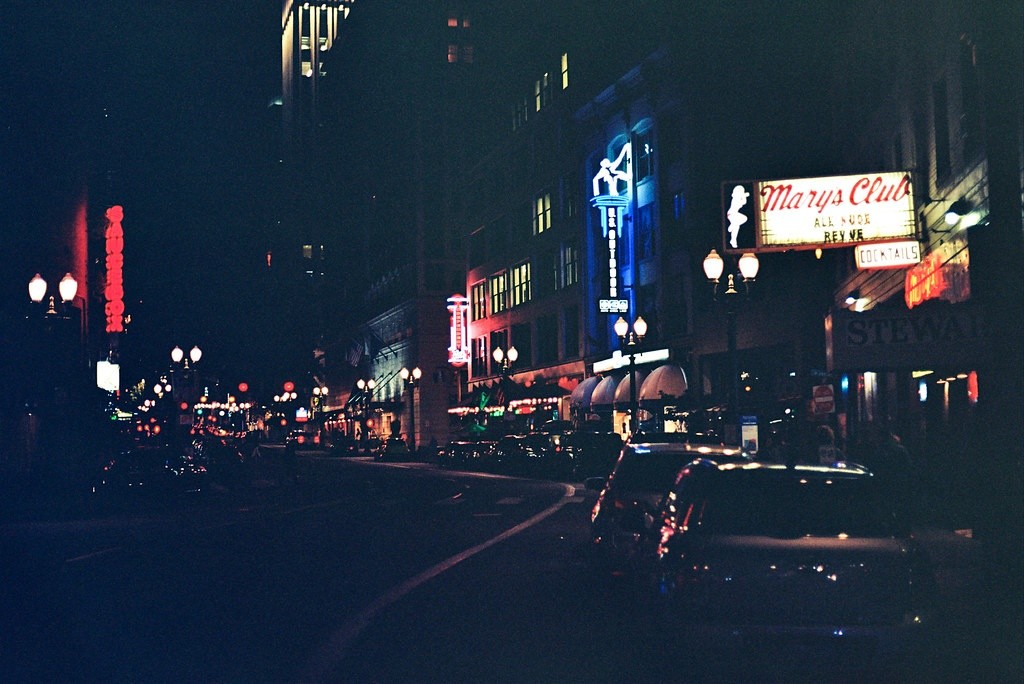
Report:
274,393,297,443
703,249,760,441
493,346,518,436
357,379,375,454
614,316,649,441
240,404,250,432
26,272,77,514
400,366,421,463
313,386,329,449
170,346,202,436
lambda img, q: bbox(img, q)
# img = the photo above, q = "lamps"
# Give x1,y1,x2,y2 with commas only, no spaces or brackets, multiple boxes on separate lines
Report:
845,286,862,305
945,197,967,226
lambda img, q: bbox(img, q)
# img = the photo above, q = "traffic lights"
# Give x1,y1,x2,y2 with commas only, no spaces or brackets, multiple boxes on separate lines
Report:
198,408,202,415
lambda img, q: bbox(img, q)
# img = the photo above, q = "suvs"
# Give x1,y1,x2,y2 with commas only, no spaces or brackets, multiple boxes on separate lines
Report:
639,456,928,671
584,435,757,594
474,435,553,477
375,439,411,462
544,430,617,480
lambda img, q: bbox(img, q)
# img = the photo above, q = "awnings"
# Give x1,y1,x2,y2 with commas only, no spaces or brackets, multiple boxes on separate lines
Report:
569,362,711,406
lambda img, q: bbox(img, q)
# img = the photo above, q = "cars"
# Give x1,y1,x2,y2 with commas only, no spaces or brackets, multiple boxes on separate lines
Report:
436,441,480,467
286,430,319,450
